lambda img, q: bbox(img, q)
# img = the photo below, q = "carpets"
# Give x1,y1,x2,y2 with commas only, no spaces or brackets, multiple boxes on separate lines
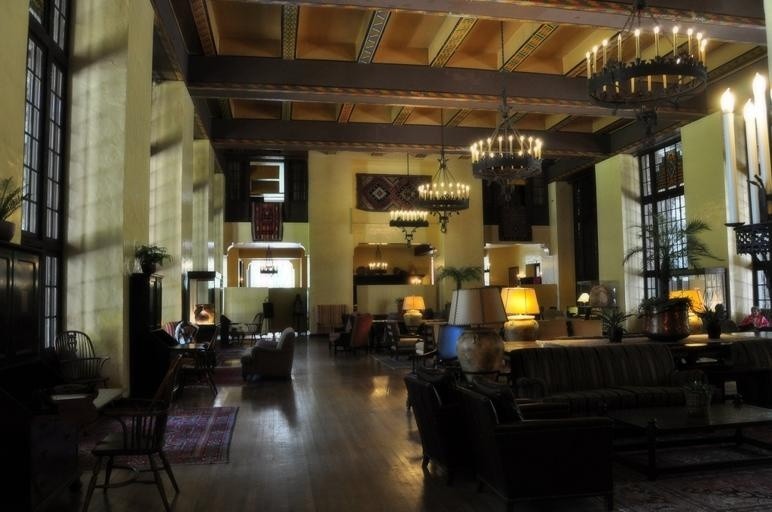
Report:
179,368,287,385
365,346,437,374
222,354,247,360
514,431,772,512
72,407,240,469
216,348,246,353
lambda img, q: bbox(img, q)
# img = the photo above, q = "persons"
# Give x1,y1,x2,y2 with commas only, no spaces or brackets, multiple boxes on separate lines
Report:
738,306,770,328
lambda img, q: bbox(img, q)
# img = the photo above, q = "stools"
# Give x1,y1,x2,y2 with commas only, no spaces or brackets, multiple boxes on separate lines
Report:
241,347,256,381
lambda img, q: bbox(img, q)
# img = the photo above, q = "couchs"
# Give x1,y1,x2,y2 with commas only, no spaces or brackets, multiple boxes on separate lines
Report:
508,342,717,442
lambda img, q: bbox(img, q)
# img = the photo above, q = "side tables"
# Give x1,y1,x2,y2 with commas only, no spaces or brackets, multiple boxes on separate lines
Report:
168,343,218,400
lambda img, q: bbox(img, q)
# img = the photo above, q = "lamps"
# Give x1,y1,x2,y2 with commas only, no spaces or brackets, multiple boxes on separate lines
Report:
448,286,510,374
390,152,428,248
402,296,426,330
586,0,707,133
500,287,541,341
368,246,389,274
417,108,470,232
470,22,543,203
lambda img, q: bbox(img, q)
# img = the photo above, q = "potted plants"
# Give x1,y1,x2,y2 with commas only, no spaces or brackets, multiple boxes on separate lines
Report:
0,177,34,247
596,310,636,343
134,245,172,275
681,381,715,416
622,198,726,340
691,304,736,338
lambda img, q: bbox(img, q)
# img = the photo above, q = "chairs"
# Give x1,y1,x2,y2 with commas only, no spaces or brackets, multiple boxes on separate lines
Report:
54,331,110,388
454,378,616,512
246,327,295,385
384,320,424,361
194,325,222,366
245,312,265,346
404,367,456,467
329,313,373,359
82,354,183,512
